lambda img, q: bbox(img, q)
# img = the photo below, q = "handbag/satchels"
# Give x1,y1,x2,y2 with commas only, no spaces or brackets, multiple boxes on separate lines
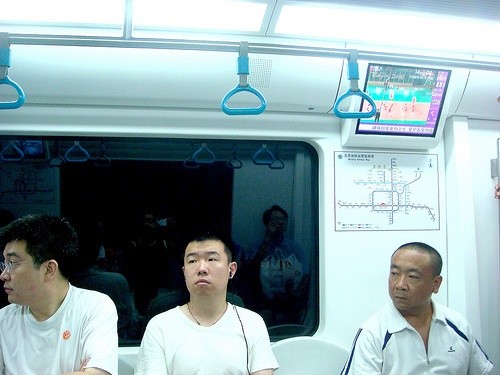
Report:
260,247,304,300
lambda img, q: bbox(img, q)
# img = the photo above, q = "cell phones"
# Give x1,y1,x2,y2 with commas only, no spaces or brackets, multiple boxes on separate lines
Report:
269,222,277,229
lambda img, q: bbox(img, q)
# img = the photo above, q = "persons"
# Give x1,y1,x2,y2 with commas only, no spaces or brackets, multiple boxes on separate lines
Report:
0,205,304,340
0,214,118,375
340,243,494,375
134,229,280,375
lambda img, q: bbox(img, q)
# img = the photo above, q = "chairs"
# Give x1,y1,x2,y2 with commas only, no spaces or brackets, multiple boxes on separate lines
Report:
271,336,351,375
267,324,312,343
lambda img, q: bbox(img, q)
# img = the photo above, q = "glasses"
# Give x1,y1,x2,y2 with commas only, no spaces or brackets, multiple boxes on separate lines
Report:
0,260,34,273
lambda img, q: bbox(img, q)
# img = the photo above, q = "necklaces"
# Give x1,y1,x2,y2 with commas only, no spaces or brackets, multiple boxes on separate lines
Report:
187,301,228,326
